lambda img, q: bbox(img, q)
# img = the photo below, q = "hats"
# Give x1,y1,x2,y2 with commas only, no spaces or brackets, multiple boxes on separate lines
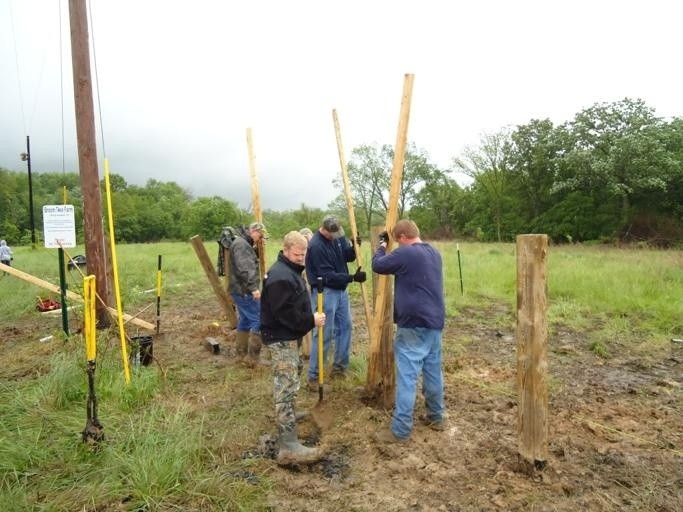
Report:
250,222,269,239
323,216,346,240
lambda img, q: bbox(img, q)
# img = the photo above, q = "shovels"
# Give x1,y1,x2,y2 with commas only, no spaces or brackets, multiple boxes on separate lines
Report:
149,255,166,339
314,278,327,408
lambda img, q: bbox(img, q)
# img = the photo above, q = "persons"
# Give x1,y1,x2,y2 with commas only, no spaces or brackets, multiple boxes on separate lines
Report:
0,240,14,276
292,227,314,394
305,215,367,392
227,223,276,368
371,219,445,445
259,230,327,467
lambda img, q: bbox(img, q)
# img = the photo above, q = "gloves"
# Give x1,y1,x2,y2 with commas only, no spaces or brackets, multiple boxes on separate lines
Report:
379,232,389,246
351,232,361,248
354,266,366,282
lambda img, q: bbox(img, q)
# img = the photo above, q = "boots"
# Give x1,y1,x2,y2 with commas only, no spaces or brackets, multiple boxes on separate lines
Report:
235,331,273,366
295,411,312,422
277,432,323,465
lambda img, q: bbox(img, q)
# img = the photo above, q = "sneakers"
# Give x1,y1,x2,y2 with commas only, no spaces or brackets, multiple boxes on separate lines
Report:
419,414,445,430
307,383,319,393
332,373,345,381
372,429,400,443
